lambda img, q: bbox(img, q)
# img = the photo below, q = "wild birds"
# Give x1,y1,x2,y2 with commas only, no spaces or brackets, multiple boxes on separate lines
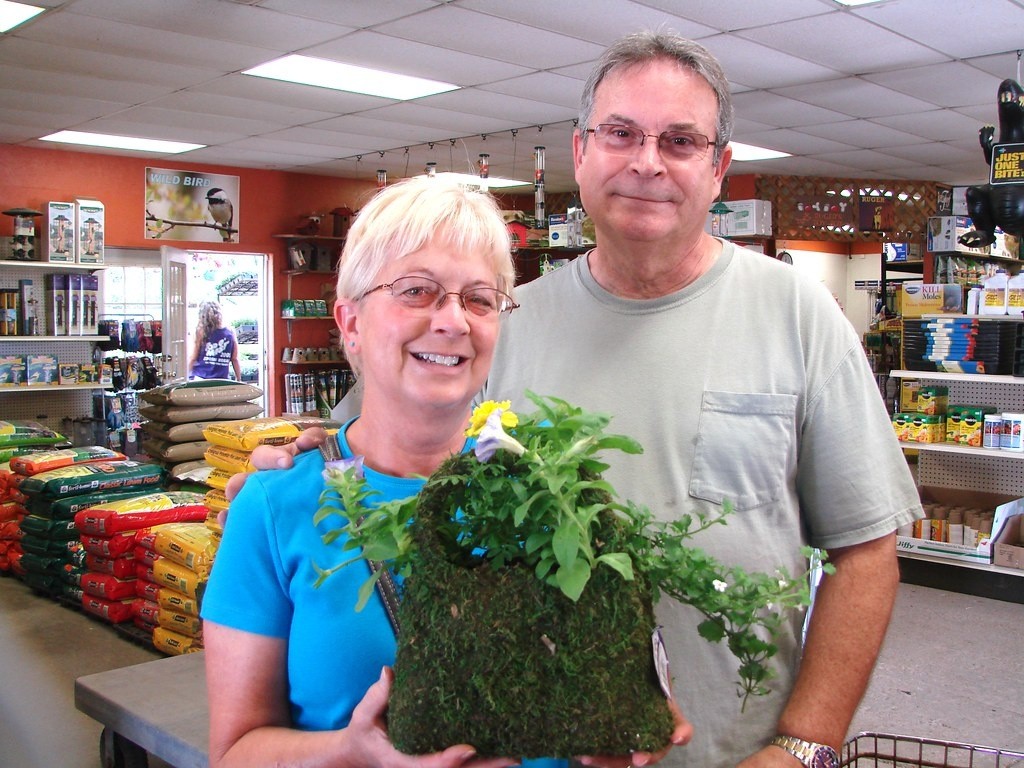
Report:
204,188,234,243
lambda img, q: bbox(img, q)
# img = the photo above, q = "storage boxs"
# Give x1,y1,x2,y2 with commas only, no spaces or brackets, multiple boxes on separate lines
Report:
707,200,773,235
45,273,99,337
0,353,113,386
547,212,585,246
280,299,327,317
72,195,105,265
867,187,1024,570
40,202,76,262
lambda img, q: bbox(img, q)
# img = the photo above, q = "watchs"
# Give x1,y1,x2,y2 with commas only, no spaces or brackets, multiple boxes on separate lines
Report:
770,735,839,768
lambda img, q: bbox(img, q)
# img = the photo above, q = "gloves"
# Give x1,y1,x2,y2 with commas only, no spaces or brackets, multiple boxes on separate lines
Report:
96,318,164,458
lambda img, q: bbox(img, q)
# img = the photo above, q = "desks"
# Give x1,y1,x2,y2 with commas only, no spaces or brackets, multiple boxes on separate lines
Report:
71,649,209,768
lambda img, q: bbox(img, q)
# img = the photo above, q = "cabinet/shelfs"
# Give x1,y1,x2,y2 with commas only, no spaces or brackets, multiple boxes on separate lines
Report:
275,234,348,365
0,237,115,432
889,314,1024,604
922,242,1024,290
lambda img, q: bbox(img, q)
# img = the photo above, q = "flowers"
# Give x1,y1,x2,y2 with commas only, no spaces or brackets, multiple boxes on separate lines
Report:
314,389,837,751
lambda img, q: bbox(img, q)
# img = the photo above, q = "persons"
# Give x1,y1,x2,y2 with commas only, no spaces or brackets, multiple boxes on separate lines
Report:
218,31,926,768
197,173,693,768
187,300,241,382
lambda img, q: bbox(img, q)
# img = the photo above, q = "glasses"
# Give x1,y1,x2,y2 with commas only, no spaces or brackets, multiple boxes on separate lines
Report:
587,124,719,164
355,276,520,324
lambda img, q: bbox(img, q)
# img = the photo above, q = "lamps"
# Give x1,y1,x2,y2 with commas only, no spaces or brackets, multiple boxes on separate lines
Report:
709,193,735,239
